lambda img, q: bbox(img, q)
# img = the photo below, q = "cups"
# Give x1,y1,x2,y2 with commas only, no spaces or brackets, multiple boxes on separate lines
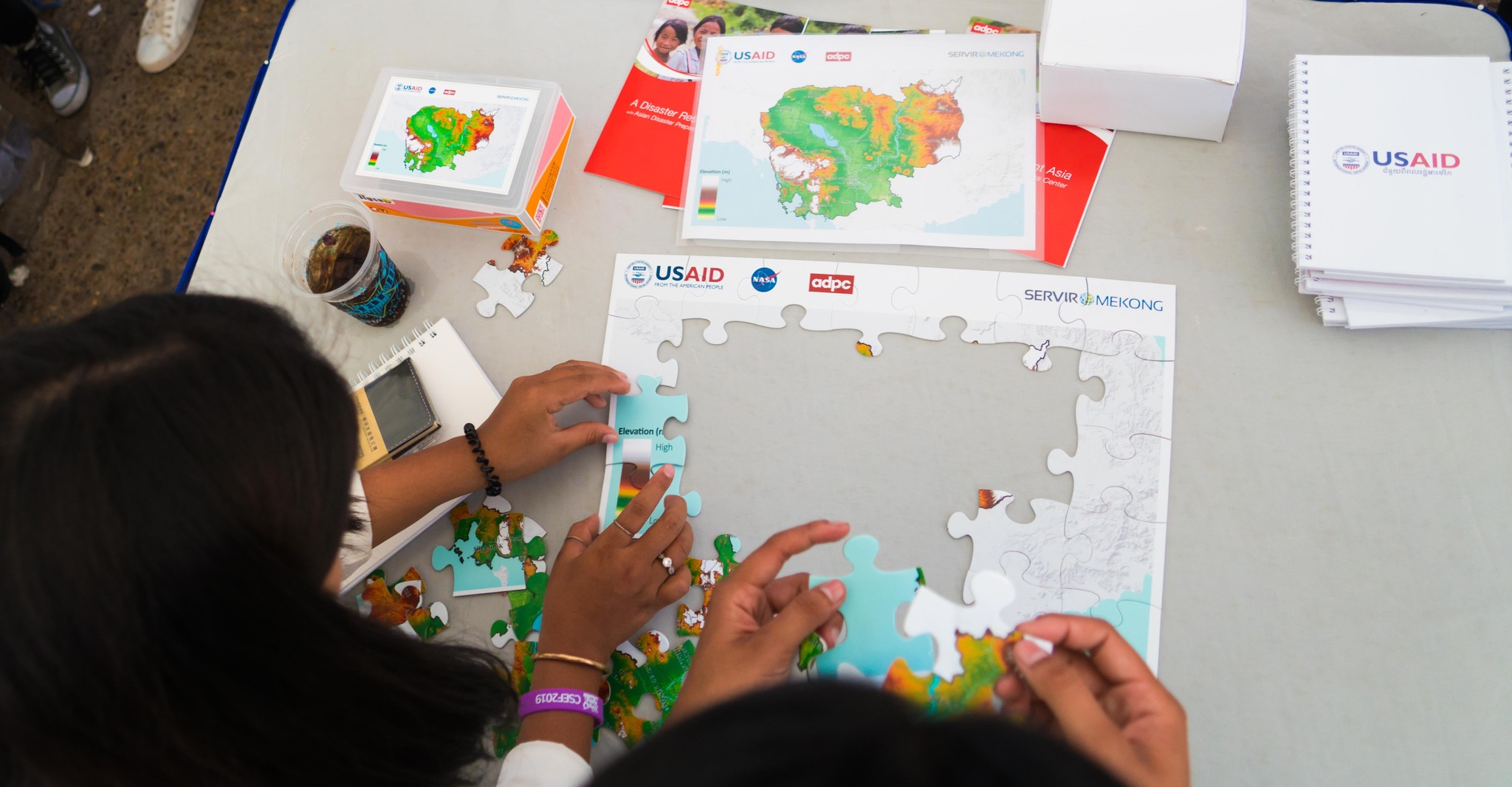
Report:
278,201,409,327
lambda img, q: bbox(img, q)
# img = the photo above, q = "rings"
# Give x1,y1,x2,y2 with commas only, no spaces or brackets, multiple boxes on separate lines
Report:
658,553,675,575
614,520,634,537
564,534,588,548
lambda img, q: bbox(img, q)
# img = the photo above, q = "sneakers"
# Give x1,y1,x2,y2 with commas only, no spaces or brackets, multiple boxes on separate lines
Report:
4,3,90,118
136,0,204,74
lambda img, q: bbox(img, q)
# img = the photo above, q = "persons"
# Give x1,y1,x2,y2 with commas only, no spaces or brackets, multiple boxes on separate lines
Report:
136,0,204,73
770,16,867,34
583,520,1190,787
0,0,88,117
0,290,692,787
635,15,726,82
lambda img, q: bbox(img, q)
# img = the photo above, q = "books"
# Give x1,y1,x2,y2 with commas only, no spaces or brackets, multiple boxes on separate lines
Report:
1287,54,1512,329
350,356,441,473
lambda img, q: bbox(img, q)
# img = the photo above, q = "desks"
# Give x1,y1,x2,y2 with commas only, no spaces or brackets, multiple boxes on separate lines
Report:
176,0,1512,787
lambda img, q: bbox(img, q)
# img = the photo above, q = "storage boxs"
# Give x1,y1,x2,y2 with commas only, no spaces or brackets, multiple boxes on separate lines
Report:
339,69,576,236
1039,1,1246,142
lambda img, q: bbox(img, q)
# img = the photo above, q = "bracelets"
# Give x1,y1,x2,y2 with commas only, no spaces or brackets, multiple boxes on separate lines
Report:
464,423,502,496
518,688,603,730
530,652,609,675
